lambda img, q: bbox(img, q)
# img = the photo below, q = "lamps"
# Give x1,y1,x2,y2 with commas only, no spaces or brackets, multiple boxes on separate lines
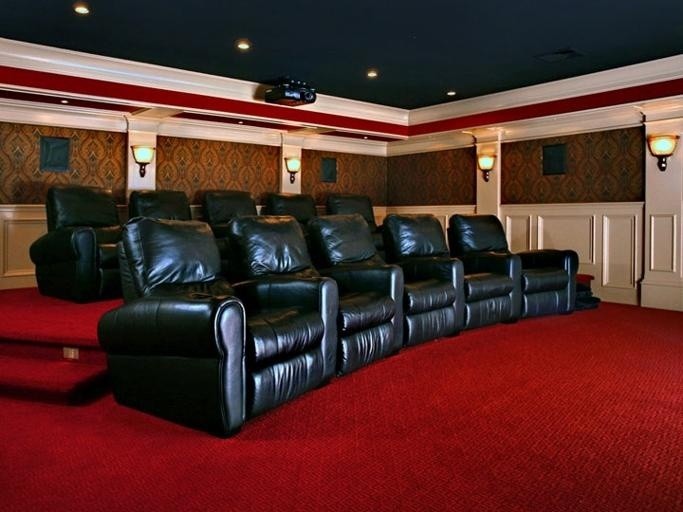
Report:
130,145,158,178
648,134,681,171
477,154,497,182
285,157,302,184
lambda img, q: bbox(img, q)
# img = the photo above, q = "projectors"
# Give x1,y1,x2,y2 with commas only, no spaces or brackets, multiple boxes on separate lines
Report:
261,84,318,109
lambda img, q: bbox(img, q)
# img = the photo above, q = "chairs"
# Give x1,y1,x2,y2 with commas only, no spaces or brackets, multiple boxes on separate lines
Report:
218,215,405,377
383,214,520,327
305,213,464,352
30,186,123,304
208,188,258,225
125,190,203,220
98,218,338,436
326,193,383,234
263,194,318,223
444,215,578,321
